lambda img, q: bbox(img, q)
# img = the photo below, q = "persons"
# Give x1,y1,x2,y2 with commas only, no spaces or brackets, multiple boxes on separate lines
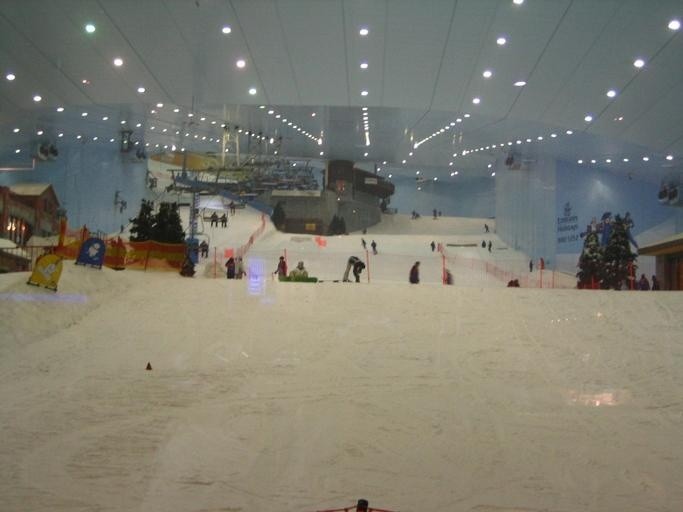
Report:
638,273,649,291
651,274,661,290
350,256,365,282
289,261,309,277
221,214,228,228
407,261,421,282
361,207,493,254
274,256,286,278
228,200,236,215
224,256,236,278
199,240,208,258
209,211,219,227
528,260,534,273
235,256,247,279
540,257,547,270
